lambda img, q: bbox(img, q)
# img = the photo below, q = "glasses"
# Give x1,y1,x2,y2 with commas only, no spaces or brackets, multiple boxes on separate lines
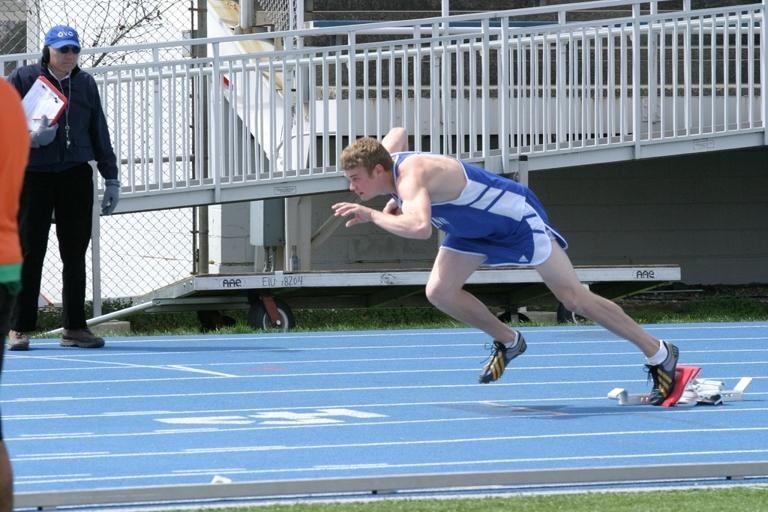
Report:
61,46,81,53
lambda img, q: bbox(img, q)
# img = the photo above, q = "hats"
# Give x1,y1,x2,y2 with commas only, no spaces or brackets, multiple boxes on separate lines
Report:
44,25,80,49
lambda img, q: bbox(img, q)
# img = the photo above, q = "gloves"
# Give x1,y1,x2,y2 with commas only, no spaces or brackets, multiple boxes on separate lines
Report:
31,115,59,148
101,180,120,215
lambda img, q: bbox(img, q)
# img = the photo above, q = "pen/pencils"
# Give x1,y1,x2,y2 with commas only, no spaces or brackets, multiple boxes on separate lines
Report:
32,118,55,120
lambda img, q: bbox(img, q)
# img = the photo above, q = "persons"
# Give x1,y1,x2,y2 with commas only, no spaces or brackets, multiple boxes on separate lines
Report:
329,124,679,406
0,75,32,512
9,23,121,349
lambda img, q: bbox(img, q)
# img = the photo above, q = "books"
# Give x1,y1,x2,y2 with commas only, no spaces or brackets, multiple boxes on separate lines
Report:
21,76,68,134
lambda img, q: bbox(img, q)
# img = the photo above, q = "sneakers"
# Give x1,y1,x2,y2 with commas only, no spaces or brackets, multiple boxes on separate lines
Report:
479,330,527,383
61,324,104,348
644,341,679,406
7,329,29,351
